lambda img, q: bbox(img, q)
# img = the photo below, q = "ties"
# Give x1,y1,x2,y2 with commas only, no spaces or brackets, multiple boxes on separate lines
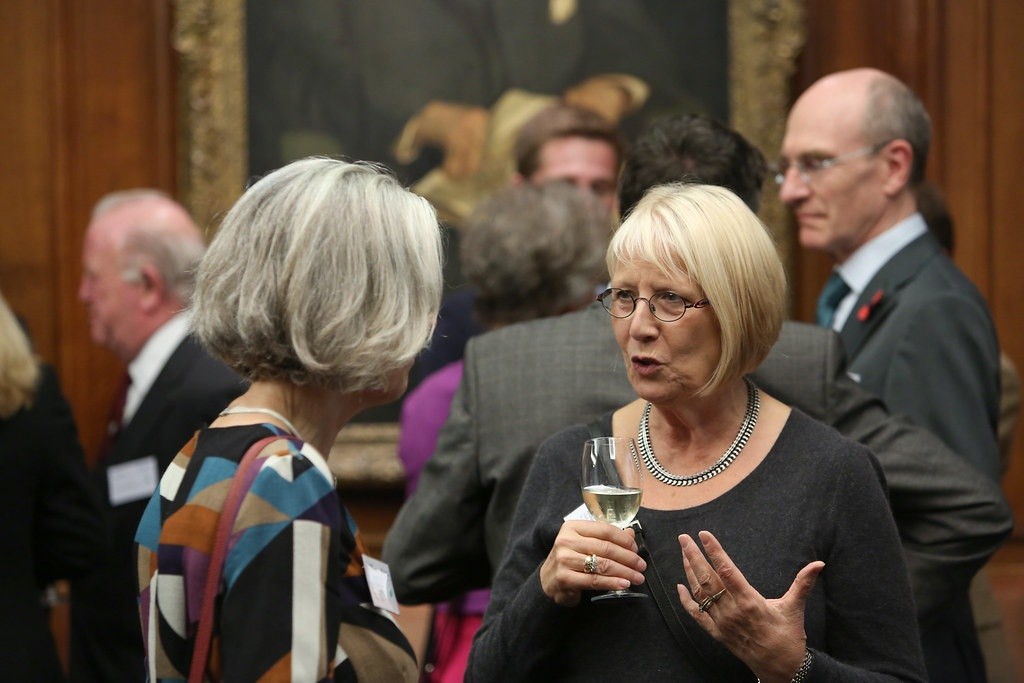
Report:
815,272,851,327
97,370,132,465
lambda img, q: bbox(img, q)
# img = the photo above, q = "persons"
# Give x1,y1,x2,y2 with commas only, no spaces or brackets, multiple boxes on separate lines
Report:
0,66,1022,683
464,183,931,683
132,155,446,683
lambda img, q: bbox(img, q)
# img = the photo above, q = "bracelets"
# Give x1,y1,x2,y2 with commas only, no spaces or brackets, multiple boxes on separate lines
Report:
757,649,812,683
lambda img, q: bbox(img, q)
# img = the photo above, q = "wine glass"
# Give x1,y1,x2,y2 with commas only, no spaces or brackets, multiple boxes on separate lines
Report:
579,436,649,603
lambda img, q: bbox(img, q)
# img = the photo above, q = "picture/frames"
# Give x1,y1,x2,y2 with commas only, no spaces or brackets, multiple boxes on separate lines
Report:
176,0,802,481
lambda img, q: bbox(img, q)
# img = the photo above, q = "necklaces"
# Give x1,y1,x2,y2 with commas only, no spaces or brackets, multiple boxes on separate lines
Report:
637,374,760,487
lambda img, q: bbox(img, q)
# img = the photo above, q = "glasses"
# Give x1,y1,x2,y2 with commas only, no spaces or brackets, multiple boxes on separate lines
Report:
596,288,710,322
770,141,891,188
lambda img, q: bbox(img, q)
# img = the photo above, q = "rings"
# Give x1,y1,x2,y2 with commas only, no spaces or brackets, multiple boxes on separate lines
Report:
700,588,726,612
583,554,593,573
591,554,597,573
